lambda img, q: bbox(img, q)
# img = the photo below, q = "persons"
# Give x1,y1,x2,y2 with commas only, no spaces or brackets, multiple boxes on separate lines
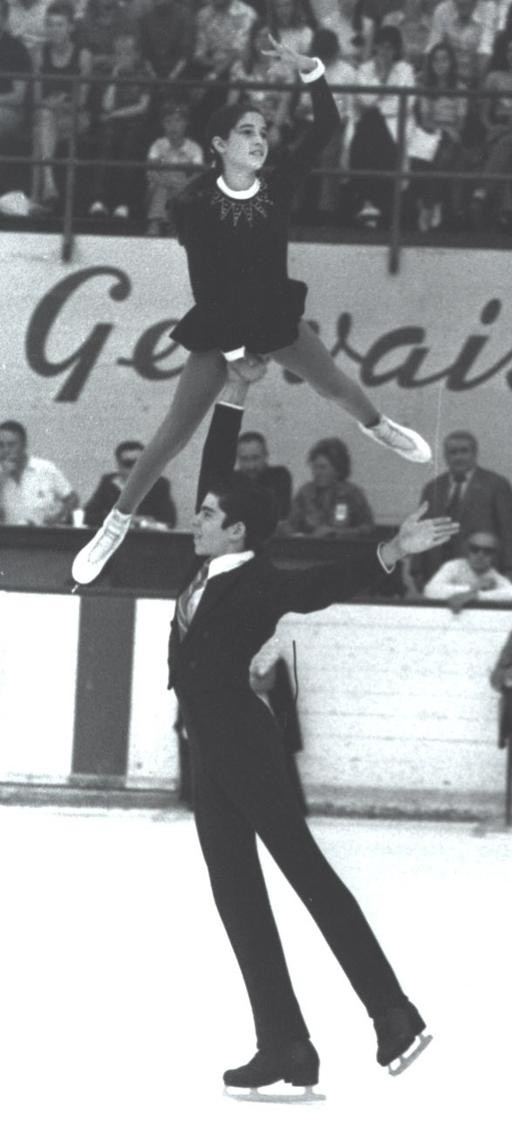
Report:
165,350,462,1088
81,436,177,529
0,419,80,526
68,31,433,589
265,434,376,537
231,430,292,533
406,427,512,594
1,0,512,230
420,528,512,616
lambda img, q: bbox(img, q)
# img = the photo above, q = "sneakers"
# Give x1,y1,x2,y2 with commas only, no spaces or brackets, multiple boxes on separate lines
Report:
376,999,427,1066
224,1043,320,1088
356,414,430,466
69,506,139,586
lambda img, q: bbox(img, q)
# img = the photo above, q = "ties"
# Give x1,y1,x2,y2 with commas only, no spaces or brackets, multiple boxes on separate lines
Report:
177,562,209,643
447,476,468,509
